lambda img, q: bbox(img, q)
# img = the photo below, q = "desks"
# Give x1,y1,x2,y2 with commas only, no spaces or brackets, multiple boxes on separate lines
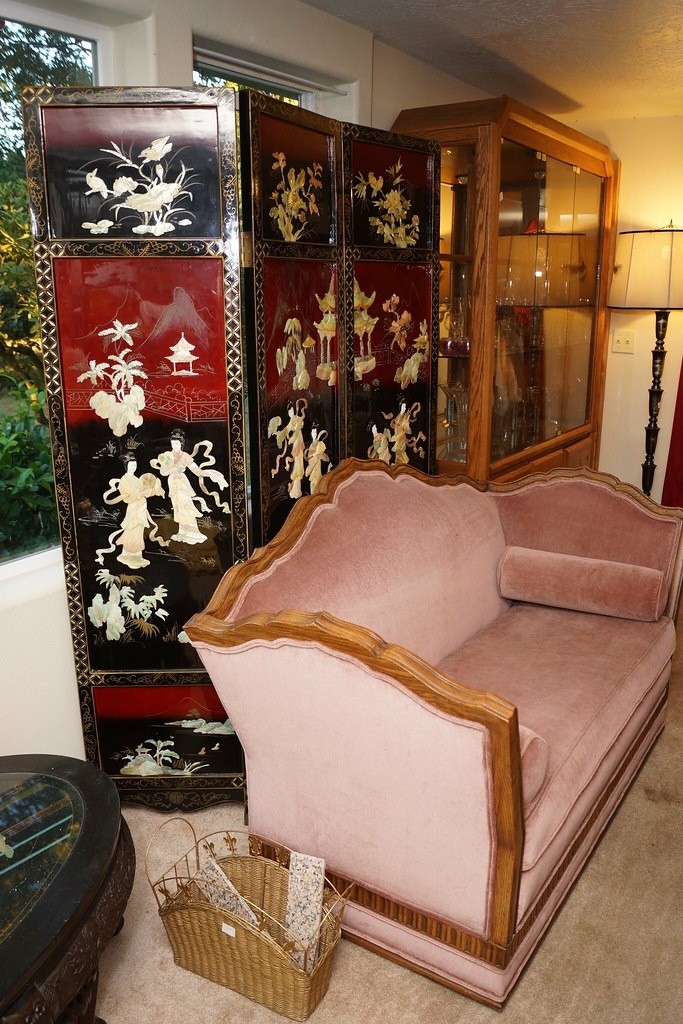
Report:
0,754,136,1024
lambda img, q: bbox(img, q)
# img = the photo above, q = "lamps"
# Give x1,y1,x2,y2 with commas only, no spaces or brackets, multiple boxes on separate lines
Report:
496,232,593,434
604,225,683,495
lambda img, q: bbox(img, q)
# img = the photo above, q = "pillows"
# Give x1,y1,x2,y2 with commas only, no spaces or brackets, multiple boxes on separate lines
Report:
517,725,549,806
496,545,669,622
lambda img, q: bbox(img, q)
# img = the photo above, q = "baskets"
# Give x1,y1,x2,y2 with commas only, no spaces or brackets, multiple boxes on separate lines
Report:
145,817,357,1022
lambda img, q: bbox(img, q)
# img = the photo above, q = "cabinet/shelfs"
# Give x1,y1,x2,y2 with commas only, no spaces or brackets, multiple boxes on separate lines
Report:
389,95,622,481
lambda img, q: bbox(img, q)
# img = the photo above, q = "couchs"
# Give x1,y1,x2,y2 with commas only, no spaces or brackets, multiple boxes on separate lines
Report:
181,455,683,1012
